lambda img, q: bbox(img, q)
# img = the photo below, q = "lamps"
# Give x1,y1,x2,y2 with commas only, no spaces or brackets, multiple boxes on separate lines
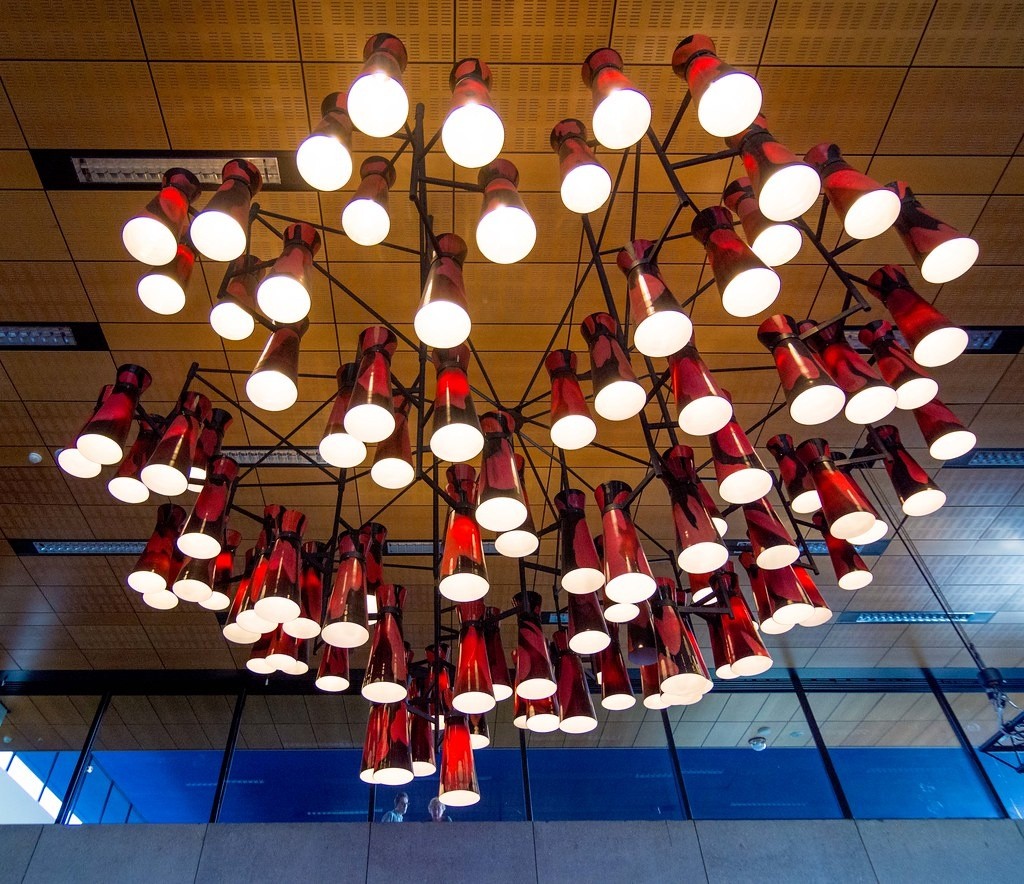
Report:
58,36,979,808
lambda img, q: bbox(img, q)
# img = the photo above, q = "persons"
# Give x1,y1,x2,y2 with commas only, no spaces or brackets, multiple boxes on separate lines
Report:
381,792,408,822
428,797,452,822
